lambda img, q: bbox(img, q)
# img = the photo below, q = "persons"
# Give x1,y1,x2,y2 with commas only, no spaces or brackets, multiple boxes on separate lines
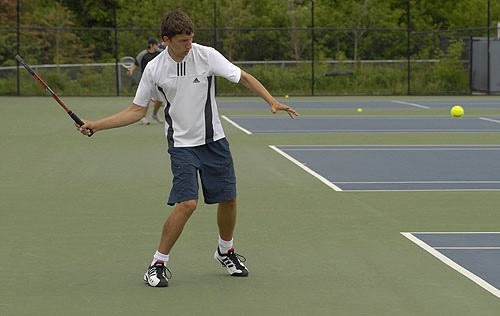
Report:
126,38,165,125
75,10,300,288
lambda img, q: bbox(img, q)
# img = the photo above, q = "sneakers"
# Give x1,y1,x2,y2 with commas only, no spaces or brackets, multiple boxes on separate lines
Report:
151,113,163,122
143,260,172,288
139,118,150,125
213,245,249,277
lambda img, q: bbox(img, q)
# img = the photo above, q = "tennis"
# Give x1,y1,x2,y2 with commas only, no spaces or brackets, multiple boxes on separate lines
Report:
357,108,363,112
450,105,464,119
285,95,289,99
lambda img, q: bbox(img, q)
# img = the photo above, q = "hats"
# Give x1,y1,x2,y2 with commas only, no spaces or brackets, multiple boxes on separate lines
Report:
148,38,160,43
